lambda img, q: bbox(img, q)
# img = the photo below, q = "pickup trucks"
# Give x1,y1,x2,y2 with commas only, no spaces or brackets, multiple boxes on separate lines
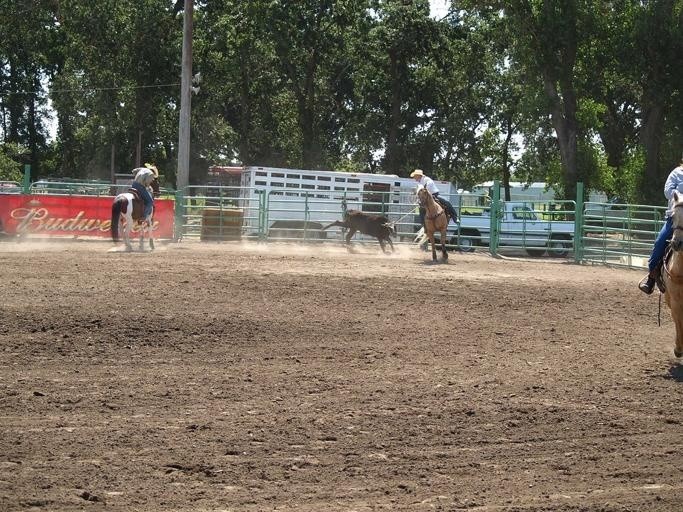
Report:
432,202,574,258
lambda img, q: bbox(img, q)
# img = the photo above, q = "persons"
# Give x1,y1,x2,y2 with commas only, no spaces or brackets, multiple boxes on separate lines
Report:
410,168,460,232
640,156,683,292
131,161,158,223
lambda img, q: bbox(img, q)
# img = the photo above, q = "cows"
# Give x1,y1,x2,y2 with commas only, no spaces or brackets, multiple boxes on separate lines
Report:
321,202,399,256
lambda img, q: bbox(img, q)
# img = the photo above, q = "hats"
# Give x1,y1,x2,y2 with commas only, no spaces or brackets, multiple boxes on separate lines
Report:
410,168,425,178
143,162,158,178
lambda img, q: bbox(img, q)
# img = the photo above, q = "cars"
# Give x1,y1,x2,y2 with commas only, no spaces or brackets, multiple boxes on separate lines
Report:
0,178,111,194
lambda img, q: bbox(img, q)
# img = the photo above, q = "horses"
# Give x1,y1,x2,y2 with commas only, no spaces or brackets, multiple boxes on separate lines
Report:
109,174,160,253
658,191,683,369
412,181,451,264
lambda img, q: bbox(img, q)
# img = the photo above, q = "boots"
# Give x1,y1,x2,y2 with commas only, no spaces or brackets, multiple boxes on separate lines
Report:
640,276,656,294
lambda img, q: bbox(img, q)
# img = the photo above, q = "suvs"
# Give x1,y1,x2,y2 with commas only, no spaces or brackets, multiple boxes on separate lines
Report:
586,193,629,236
